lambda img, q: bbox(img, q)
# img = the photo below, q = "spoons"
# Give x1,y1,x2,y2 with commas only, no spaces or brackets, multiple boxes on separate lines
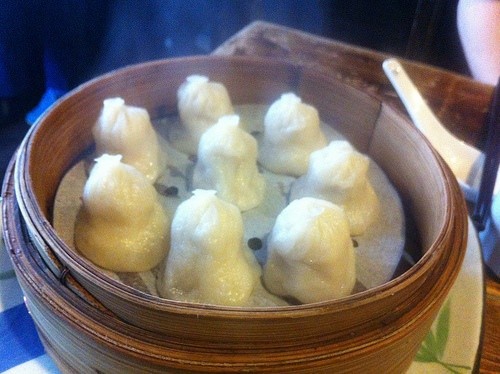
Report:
383,57,486,203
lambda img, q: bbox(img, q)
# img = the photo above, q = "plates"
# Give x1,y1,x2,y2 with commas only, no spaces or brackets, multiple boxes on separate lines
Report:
1,216,484,373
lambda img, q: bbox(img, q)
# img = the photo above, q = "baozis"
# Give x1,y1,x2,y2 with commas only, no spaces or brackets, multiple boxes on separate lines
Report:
260,196,357,304
157,189,264,307
75,153,169,272
262,93,324,176
191,115,268,212
167,74,235,150
87,98,163,185
288,140,380,237
